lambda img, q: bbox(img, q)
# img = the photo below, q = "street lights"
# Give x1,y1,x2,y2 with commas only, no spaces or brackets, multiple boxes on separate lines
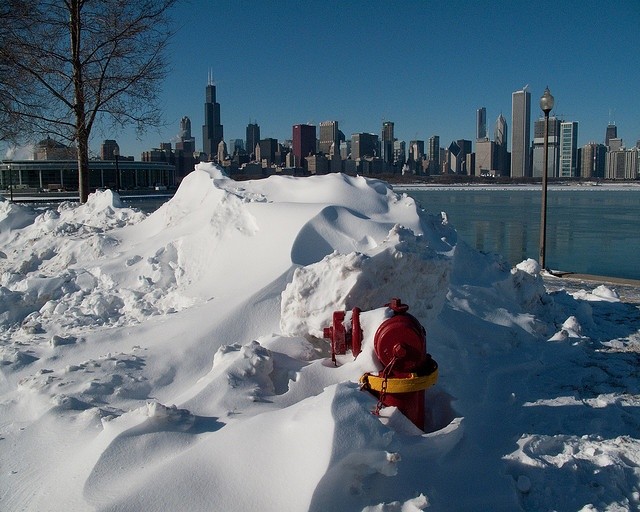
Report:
539,86,554,269
113,146,120,195
8,164,13,204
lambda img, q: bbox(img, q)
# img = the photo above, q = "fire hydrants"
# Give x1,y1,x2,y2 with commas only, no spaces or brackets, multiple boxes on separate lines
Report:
325,296,439,435
